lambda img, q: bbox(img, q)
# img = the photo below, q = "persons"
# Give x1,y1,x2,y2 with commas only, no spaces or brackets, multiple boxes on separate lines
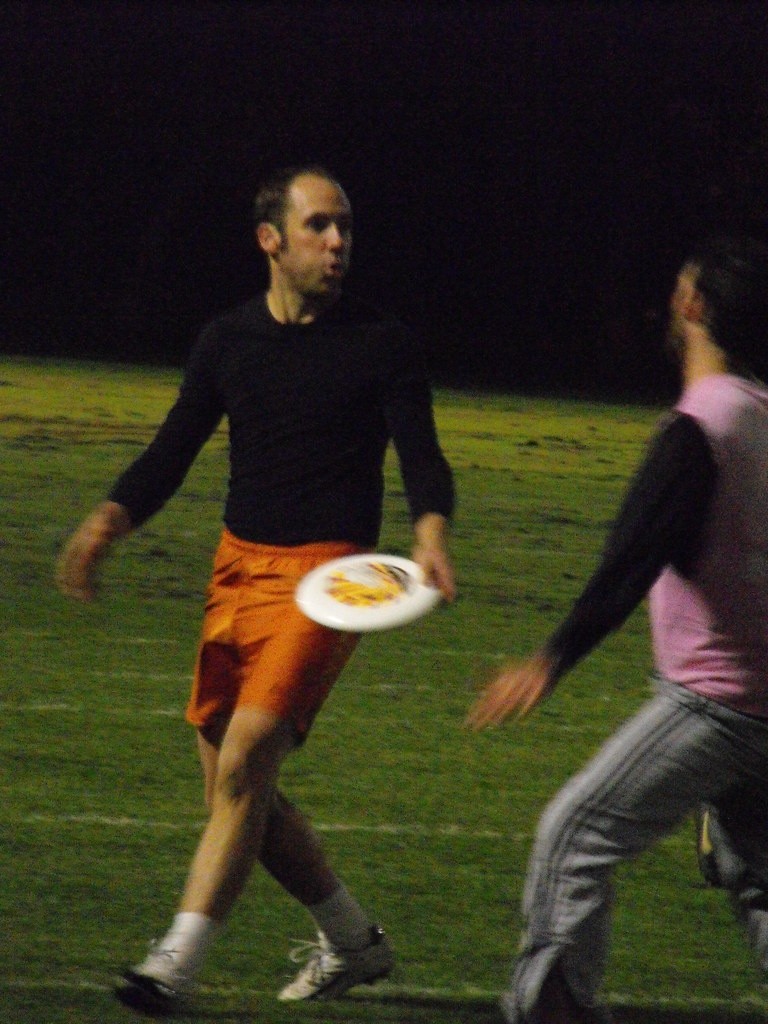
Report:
57,158,459,1014
465,240,768,1024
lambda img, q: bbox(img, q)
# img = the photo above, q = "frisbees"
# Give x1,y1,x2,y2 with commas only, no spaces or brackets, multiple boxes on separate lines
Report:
293,551,442,632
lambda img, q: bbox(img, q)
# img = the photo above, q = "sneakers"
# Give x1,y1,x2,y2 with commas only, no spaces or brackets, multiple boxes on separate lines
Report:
110,942,195,1013
275,922,399,1008
